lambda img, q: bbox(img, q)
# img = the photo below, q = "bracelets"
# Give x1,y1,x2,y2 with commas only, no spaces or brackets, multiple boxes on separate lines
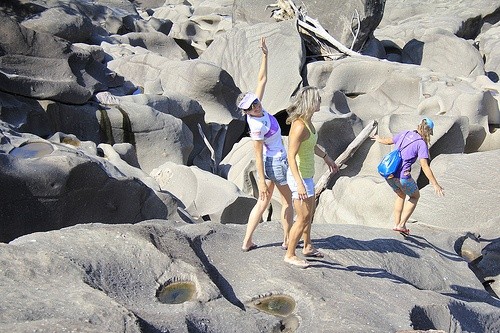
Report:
322,154,327,158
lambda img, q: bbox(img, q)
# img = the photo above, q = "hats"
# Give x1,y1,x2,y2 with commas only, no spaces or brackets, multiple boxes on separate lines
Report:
238,92,258,109
420,117,434,136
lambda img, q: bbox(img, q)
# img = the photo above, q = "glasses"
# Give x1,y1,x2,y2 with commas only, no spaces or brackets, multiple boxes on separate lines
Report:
318,97,321,103
248,99,259,111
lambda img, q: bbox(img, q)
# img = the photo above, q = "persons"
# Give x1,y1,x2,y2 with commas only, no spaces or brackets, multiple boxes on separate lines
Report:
283,86,341,268
236,36,300,252
370,118,445,233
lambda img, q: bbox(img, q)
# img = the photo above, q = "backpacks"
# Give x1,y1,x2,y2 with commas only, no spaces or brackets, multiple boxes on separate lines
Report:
377,131,423,179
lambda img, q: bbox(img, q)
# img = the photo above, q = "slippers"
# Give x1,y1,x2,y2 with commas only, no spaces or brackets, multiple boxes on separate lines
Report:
392,227,409,235
285,258,311,268
304,251,324,257
241,243,257,252
281,246,288,250
